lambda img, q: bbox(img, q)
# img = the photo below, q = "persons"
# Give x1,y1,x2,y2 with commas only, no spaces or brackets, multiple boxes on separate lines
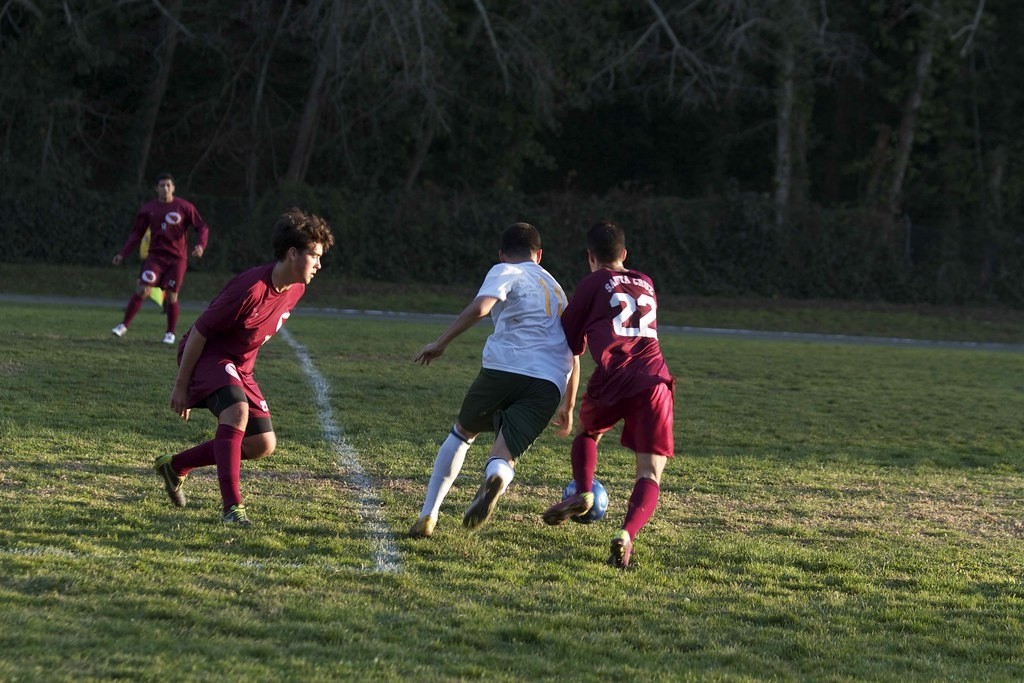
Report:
112,173,208,344
155,209,334,527
408,223,574,539
561,219,675,570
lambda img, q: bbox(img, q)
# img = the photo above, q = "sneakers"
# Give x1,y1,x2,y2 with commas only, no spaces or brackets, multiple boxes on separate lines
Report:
220,495,252,526
607,529,635,568
543,492,594,525
156,453,186,508
111,323,127,337
162,331,176,344
408,515,437,537
462,473,505,532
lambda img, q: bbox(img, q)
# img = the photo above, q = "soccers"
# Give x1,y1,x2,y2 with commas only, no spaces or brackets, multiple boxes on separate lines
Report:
562,478,609,524
